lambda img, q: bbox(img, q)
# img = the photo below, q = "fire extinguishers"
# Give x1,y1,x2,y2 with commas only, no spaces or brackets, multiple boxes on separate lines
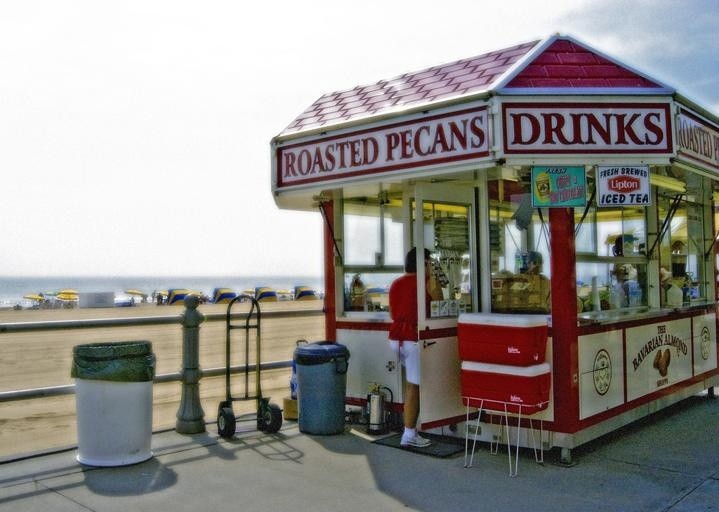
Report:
366,383,393,434
350,274,365,311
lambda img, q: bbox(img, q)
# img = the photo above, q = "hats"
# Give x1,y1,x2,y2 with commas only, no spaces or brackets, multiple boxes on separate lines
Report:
615,234,638,245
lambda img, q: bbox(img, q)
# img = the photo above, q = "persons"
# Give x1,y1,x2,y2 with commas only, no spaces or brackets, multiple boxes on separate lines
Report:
528,250,542,274
387,245,444,447
609,233,636,310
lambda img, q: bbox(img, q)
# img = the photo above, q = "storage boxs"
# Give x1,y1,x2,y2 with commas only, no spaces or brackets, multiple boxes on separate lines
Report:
458,314,548,367
283,396,297,420
462,362,550,414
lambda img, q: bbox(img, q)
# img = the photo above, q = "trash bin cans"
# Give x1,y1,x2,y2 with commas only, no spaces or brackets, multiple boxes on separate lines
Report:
71,340,155,467
295,341,350,435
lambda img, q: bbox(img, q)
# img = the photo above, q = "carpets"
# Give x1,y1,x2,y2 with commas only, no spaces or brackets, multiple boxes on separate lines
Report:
371,434,466,458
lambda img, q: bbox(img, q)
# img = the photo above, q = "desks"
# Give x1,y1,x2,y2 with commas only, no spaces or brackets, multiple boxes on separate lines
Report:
462,397,544,477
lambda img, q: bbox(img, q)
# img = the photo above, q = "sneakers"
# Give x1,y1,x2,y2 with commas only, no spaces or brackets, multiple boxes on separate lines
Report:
400,434,433,449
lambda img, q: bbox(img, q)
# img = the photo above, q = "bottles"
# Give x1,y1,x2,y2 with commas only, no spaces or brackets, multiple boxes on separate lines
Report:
353,277,366,311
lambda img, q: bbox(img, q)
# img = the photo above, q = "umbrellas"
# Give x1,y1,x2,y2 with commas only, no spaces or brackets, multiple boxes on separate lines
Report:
125,288,142,295
23,288,79,310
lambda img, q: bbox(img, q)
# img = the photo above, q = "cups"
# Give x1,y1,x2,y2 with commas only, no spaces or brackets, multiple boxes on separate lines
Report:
535,176,551,199
